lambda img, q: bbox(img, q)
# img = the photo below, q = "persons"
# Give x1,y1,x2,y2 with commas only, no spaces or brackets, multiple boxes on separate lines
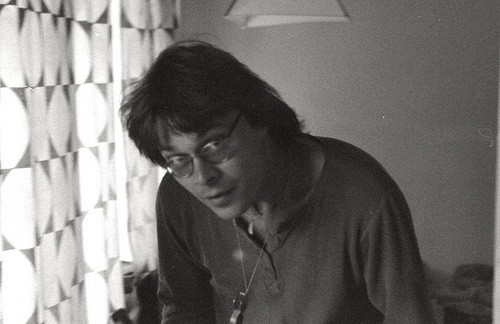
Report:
117,38,436,324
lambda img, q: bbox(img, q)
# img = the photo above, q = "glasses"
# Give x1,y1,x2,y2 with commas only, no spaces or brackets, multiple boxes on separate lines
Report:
163,111,242,177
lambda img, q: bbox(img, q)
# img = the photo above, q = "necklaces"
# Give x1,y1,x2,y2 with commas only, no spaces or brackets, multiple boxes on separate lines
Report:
230,139,294,324
